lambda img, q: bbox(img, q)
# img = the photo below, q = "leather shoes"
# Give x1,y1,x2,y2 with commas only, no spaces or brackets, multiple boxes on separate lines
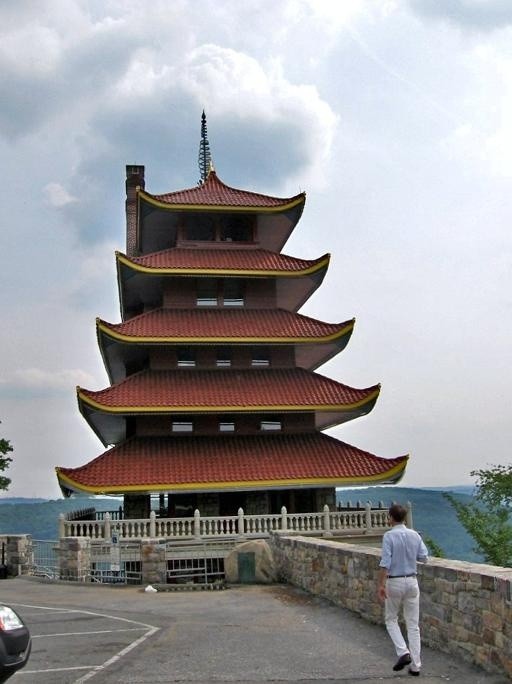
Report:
408,667,420,676
393,653,412,671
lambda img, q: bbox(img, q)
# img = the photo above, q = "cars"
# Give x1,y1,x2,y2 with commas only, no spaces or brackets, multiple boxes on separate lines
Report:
0,603,33,684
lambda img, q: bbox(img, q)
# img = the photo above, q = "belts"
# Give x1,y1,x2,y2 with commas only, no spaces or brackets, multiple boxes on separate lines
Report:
388,573,416,577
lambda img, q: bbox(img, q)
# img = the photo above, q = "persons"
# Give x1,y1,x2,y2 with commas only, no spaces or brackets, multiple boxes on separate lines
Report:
376,505,429,677
